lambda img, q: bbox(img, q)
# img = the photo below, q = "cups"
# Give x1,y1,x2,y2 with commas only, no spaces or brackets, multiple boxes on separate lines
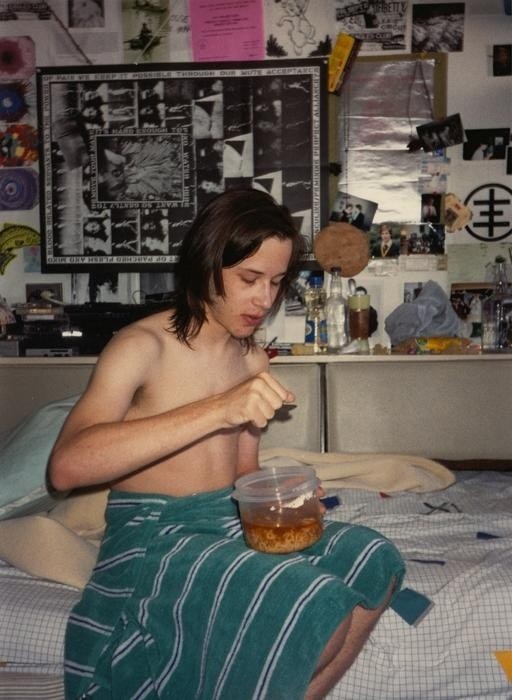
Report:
348,294,370,352
231,463,324,553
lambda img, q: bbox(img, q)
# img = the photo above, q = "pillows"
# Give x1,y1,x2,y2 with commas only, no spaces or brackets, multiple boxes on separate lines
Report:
0,393,107,520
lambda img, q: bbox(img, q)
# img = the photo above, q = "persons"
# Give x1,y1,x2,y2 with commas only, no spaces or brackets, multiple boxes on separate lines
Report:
399,224,441,254
49,81,280,243
373,225,398,258
471,142,493,160
422,124,441,150
355,286,378,338
350,204,365,226
46,186,406,698
363,4,380,29
422,197,437,223
330,200,346,222
439,126,455,147
340,204,352,224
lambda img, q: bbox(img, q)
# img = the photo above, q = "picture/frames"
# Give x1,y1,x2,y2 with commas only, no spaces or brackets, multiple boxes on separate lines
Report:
35,57,328,273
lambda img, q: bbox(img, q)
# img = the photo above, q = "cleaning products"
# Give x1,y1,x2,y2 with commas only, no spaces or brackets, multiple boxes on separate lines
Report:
306,278,329,352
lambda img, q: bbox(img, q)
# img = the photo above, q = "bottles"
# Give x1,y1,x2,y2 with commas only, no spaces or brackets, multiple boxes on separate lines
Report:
480,254,511,351
324,265,350,350
302,275,327,353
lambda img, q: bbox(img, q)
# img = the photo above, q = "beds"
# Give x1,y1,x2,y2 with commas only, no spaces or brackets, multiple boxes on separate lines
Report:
0,355,512,700
